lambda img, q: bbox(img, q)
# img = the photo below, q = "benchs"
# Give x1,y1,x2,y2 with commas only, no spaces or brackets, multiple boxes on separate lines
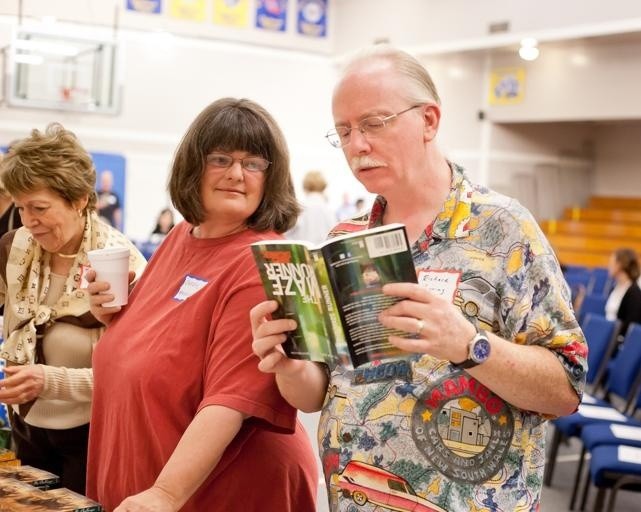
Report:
523,194,641,271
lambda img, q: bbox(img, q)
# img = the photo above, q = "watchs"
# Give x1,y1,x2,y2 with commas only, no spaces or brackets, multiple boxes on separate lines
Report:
449,320,491,370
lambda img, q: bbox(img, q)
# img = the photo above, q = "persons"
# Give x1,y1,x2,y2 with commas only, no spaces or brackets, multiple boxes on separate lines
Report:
0,149,25,318
153,206,177,233
82,93,322,512
246,47,592,512
0,120,150,496
294,165,340,240
93,168,124,229
598,247,641,405
352,197,369,218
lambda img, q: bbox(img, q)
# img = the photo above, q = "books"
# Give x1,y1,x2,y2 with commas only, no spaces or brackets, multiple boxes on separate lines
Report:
248,221,423,368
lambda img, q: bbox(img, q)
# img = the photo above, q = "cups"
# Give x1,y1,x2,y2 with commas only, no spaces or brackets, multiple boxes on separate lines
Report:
87,247,130,308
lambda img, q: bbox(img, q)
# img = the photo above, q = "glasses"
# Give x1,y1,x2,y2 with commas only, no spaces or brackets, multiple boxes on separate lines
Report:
324,106,419,148
206,153,273,171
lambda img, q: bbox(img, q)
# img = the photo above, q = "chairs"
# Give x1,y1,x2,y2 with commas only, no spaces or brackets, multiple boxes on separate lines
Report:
544,268,641,510
